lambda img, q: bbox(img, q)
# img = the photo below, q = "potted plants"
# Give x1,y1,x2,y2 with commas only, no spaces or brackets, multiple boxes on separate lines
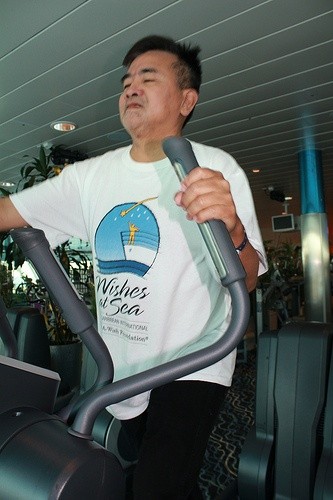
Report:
15,276,82,380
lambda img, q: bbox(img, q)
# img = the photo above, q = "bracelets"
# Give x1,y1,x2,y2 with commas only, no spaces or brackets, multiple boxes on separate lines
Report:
233,224,248,254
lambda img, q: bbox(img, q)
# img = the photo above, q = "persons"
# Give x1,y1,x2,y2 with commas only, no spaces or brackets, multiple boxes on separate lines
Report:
0,35,268,500
